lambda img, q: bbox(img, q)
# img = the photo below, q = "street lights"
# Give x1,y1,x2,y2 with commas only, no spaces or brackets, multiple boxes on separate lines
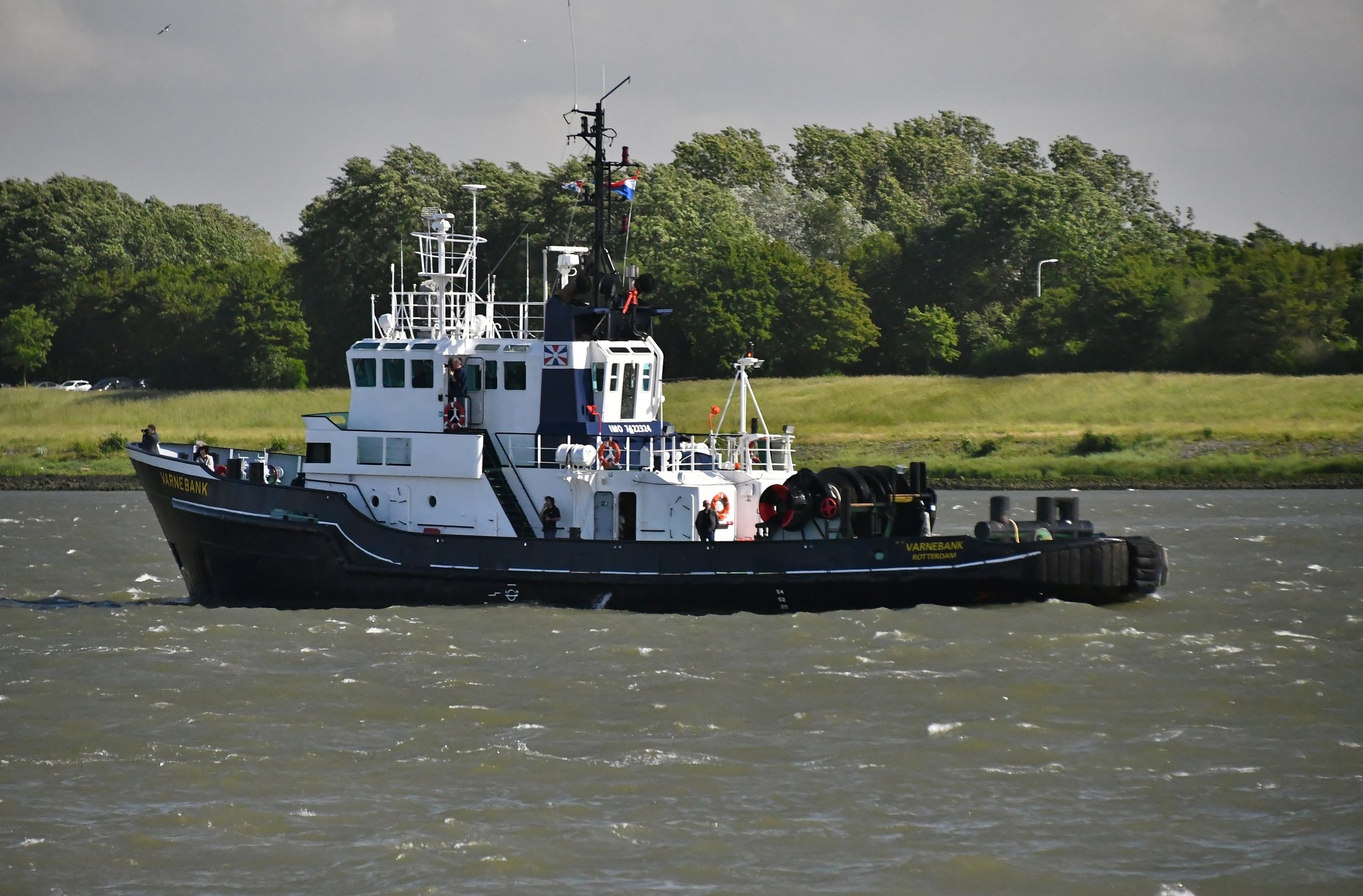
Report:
1036,258,1060,298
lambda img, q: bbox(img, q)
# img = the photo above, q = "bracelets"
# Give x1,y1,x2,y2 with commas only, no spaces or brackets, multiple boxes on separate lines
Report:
542,509,544,513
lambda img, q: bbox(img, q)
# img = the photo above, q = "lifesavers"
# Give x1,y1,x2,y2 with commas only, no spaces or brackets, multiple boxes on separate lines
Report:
711,492,730,521
443,401,465,430
748,437,766,463
598,440,621,469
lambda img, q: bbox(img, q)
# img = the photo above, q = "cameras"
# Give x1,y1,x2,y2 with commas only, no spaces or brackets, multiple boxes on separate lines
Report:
141,428,149,434
445,364,453,369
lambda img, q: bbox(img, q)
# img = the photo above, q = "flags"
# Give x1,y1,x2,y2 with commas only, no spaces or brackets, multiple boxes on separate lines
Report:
593,176,637,201
561,180,584,192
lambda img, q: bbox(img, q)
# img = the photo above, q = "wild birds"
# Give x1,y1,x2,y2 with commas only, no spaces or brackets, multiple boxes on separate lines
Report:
156,23,172,36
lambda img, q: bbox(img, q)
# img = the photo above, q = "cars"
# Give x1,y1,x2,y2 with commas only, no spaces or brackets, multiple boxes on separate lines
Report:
58,380,92,391
132,379,157,390
27,381,59,389
88,377,135,392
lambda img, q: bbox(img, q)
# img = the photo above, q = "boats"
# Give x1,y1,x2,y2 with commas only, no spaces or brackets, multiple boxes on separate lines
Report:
121,0,1168,615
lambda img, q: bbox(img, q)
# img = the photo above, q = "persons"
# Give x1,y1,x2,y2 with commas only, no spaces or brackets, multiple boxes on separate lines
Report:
695,499,719,542
448,358,468,406
539,496,561,538
196,445,215,473
142,424,159,453
